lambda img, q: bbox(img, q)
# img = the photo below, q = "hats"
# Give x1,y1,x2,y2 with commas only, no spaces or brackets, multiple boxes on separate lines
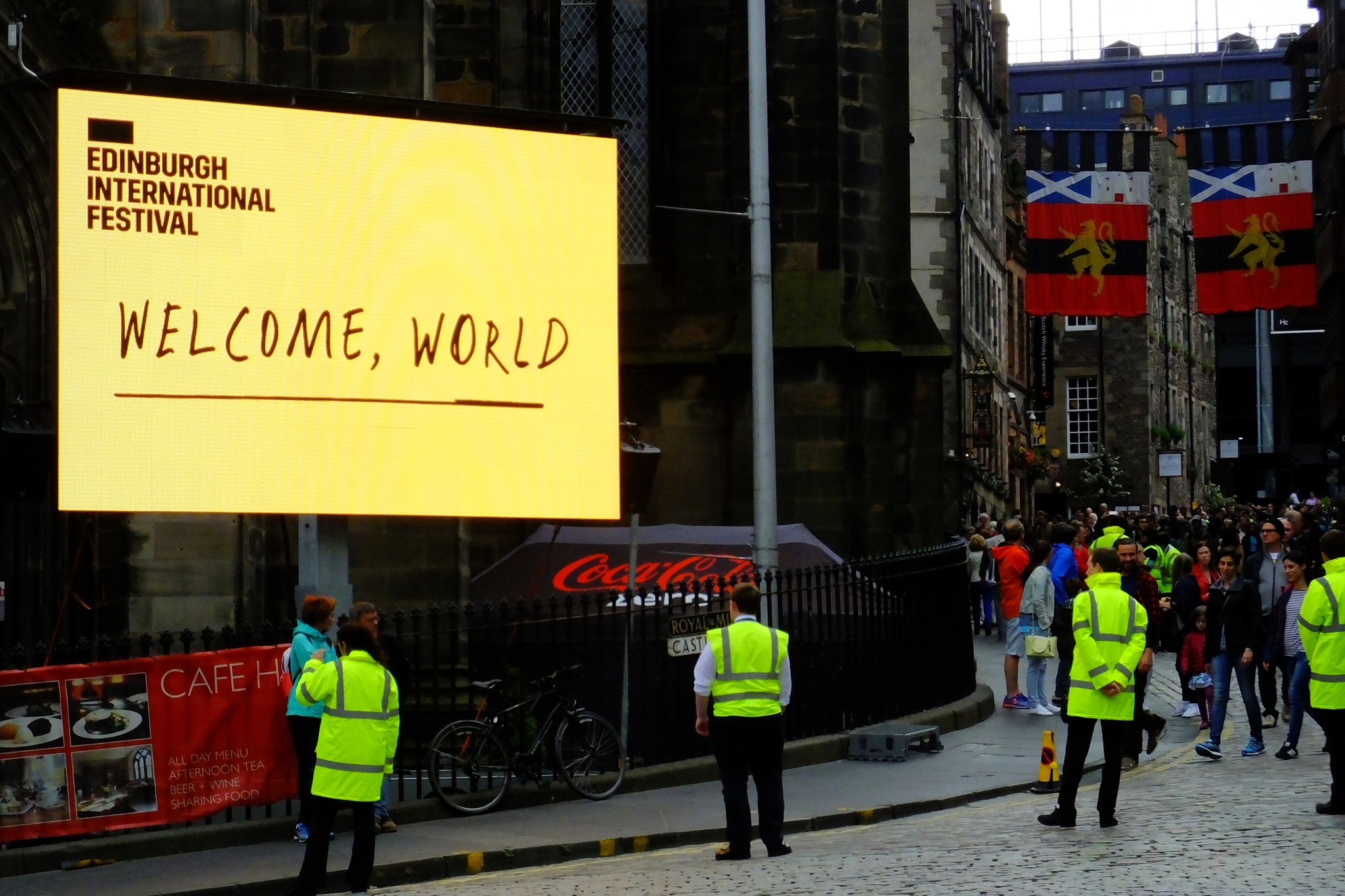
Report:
1263,517,1285,539
1192,515,1201,520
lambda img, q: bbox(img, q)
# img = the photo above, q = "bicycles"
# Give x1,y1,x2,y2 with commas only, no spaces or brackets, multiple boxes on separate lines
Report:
428,663,626,814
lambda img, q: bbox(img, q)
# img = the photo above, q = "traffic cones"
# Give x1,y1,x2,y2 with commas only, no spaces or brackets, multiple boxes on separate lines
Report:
1031,731,1063,794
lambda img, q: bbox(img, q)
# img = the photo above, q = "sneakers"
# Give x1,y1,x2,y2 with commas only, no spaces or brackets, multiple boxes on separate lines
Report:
1029,704,1054,715
330,833,337,838
1044,703,1062,712
1002,692,1030,708
294,823,308,842
1200,721,1211,729
1172,699,1330,760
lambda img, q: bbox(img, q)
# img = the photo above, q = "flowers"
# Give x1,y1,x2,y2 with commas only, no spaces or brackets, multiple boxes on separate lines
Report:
1017,446,1042,466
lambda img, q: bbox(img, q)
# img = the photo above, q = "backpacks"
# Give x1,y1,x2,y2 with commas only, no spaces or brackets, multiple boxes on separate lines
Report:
282,638,333,700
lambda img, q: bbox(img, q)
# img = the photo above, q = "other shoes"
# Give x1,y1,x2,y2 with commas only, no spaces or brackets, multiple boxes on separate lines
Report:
381,820,397,832
1315,801,1345,815
375,817,381,832
285,884,316,896
768,844,792,857
715,845,751,860
352,884,367,892
1099,816,1118,827
1000,633,1007,640
1145,713,1166,754
1121,757,1138,769
1038,805,1077,827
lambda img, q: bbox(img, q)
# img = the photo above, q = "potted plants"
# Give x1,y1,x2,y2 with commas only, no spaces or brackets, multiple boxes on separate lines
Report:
1160,428,1170,447
1149,330,1155,344
1186,464,1200,482
1204,473,1211,486
1151,425,1161,442
1184,349,1196,367
1202,361,1215,377
1158,332,1180,356
1168,421,1185,444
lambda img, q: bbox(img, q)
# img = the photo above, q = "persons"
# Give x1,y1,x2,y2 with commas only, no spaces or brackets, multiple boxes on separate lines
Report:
691,582,792,861
961,492,1345,828
348,600,397,836
285,594,338,843
272,622,400,895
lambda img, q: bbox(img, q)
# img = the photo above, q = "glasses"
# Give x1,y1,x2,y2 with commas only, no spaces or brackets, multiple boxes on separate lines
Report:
329,611,334,618
1262,528,1277,535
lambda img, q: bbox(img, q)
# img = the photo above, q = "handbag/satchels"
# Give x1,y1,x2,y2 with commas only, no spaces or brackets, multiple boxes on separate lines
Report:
1024,635,1058,658
